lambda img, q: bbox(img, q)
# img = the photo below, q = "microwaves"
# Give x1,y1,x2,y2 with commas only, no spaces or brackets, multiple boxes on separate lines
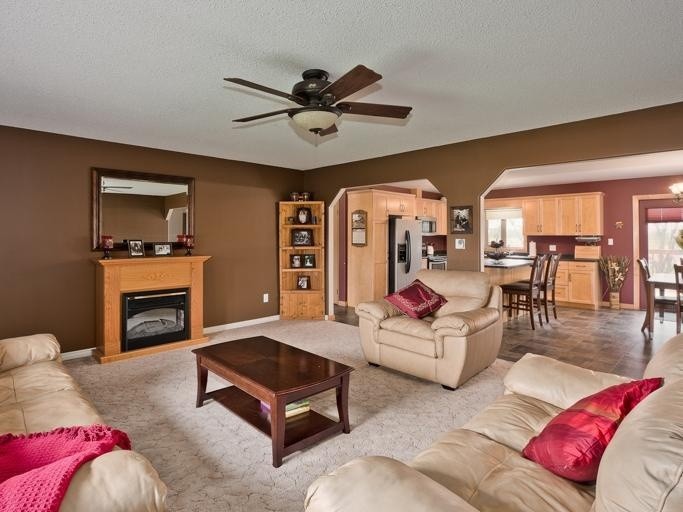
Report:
415,216,437,236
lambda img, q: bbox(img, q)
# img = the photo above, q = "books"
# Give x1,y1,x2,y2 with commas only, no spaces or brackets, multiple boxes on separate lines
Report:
261,400,311,426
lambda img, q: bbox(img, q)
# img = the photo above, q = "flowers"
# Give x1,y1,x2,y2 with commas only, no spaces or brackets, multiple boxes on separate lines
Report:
598,255,632,293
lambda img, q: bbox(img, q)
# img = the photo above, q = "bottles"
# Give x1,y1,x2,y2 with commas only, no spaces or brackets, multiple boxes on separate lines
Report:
288,192,309,201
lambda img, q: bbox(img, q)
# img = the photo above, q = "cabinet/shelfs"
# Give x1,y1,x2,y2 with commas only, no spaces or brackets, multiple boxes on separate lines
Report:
421,195,448,237
570,260,604,309
277,200,327,322
554,260,570,308
345,189,389,309
560,191,606,238
522,194,560,238
387,191,418,217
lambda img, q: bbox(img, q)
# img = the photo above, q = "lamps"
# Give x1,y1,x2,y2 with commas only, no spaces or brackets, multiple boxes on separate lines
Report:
288,107,343,136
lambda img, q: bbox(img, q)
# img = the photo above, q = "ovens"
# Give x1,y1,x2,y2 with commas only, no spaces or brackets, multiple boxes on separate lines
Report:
120,289,190,351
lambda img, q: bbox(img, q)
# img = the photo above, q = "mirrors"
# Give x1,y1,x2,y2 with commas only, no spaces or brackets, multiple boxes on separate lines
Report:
90,167,196,253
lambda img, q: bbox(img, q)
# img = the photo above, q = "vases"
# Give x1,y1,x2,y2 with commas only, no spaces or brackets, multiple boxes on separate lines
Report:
608,293,621,311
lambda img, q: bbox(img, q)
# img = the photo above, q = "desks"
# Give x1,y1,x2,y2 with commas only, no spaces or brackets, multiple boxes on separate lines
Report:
644,272,683,340
483,257,534,323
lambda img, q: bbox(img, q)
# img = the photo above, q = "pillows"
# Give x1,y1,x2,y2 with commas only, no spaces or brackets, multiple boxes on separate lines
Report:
383,277,449,320
522,374,665,487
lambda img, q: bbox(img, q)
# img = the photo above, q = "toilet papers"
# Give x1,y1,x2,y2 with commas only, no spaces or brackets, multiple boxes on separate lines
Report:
529,242,537,257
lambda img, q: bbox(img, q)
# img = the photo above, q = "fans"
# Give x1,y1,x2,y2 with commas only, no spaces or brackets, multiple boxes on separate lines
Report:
219,62,414,123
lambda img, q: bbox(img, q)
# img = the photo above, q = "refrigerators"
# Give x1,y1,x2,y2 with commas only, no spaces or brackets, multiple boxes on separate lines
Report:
388,219,421,294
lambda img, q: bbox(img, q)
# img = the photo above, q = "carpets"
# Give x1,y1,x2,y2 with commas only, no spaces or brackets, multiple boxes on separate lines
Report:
60,321,517,512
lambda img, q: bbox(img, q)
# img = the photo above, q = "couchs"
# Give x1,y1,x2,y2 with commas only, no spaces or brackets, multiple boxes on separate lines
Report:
2,332,172,512
302,328,683,512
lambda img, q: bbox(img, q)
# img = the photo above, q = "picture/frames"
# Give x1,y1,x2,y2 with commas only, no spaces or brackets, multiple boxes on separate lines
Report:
128,238,145,259
288,206,317,291
151,242,174,256
449,205,474,235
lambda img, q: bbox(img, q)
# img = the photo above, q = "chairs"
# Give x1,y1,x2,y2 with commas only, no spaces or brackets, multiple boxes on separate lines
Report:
673,263,683,335
498,251,546,331
636,256,683,334
520,251,562,323
354,268,505,392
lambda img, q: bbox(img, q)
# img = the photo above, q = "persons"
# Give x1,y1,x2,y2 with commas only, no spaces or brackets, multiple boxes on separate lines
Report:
455,212,472,234
